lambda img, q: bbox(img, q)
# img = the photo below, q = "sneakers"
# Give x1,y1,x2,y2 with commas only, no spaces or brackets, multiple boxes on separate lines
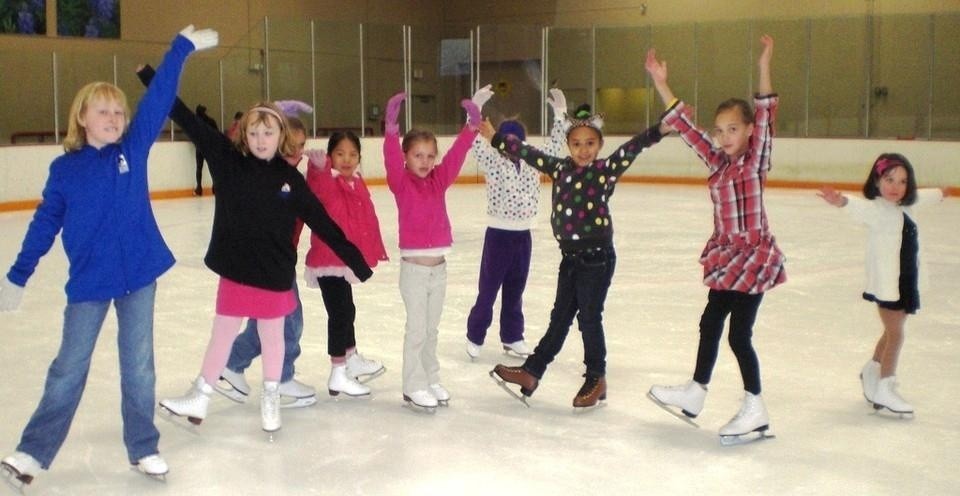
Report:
502,339,535,358
426,381,451,407
718,389,769,445
345,353,386,385
403,388,439,415
326,365,370,397
647,380,707,428
873,378,913,419
859,362,882,405
260,380,281,442
490,364,539,404
280,377,316,409
159,388,211,435
465,339,484,363
1,452,42,483
216,367,249,404
572,375,606,407
137,454,168,481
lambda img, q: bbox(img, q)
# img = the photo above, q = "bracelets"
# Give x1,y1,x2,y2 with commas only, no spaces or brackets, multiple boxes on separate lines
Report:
664,97,678,111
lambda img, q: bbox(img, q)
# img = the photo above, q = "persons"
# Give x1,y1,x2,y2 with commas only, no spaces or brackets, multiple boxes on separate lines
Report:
135,61,373,431
477,104,694,414
192,104,244,196
645,34,788,448
303,130,387,395
383,85,494,410
465,82,568,362
815,154,948,415
1,23,220,486
216,117,316,408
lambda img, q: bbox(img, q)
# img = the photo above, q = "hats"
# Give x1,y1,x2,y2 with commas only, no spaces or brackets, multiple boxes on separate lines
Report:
498,120,528,140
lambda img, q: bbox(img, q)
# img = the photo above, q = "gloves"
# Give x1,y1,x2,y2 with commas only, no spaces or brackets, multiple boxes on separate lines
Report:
546,88,567,111
460,99,480,128
303,149,327,169
472,83,494,114
386,92,406,134
0,275,24,311
274,101,313,119
179,24,219,50
